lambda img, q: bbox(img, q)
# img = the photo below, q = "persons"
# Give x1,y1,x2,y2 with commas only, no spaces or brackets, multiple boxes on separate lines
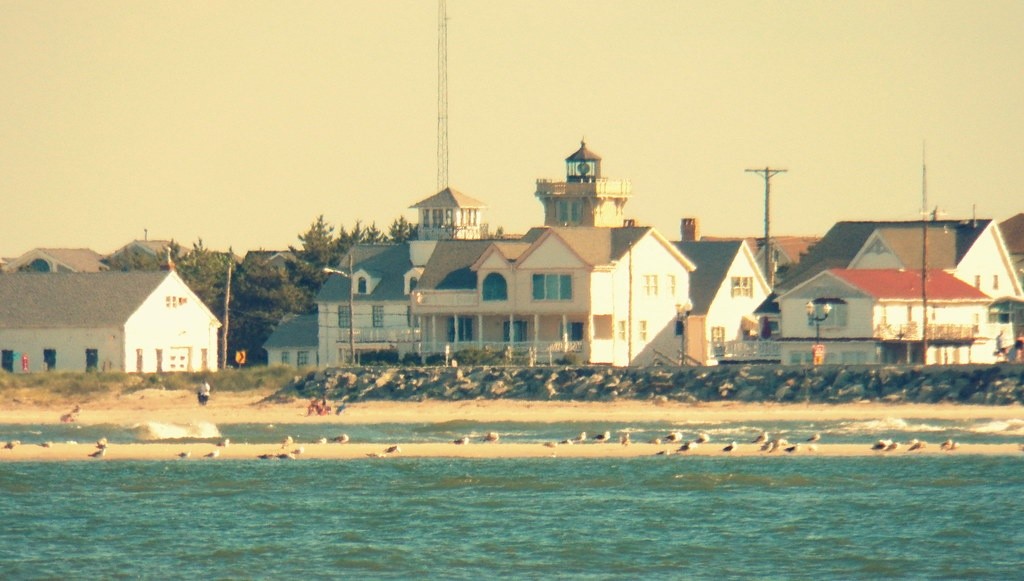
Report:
197,378,211,405
1011,332,1024,361
996,329,1008,362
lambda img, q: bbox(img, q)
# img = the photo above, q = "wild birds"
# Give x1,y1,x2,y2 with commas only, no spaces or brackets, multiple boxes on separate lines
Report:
176,431,500,459
542,427,959,454
4,437,110,461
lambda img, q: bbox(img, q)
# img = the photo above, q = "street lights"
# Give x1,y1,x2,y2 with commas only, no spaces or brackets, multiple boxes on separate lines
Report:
805,300,832,366
322,254,358,365
